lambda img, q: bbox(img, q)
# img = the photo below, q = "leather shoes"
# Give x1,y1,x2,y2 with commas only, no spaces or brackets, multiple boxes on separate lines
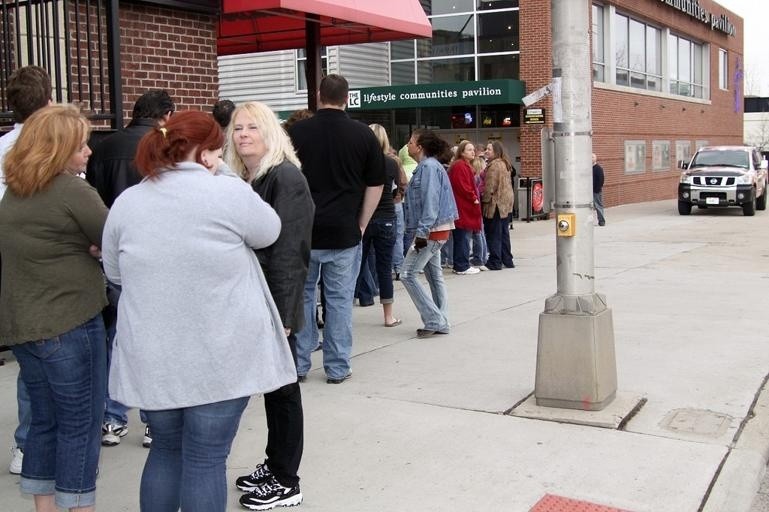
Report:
312,342,324,353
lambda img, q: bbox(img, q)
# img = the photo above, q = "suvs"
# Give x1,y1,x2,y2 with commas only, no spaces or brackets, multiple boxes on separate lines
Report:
677,145,768,216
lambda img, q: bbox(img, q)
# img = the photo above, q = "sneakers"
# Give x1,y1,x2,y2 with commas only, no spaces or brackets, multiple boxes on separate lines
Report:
9,445,23,475
102,421,128,446
239,474,303,510
143,422,152,448
236,459,272,492
480,266,491,270
417,322,446,338
327,367,353,384
456,266,480,274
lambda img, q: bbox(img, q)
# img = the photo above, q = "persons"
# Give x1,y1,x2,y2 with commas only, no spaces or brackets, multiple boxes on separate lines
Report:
357,123,401,326
225,102,315,510
403,129,458,338
0,65,88,476
592,153,605,226
213,97,235,129
355,258,379,307
102,111,297,512
292,74,385,385
439,136,515,274
398,142,419,252
90,90,175,447
388,147,405,283
0,102,110,512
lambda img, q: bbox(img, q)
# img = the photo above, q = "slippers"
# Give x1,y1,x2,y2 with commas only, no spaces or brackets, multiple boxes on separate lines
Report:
385,319,402,327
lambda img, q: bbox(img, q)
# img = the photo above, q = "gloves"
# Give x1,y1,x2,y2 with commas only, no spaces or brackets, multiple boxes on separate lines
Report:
414,237,427,253
